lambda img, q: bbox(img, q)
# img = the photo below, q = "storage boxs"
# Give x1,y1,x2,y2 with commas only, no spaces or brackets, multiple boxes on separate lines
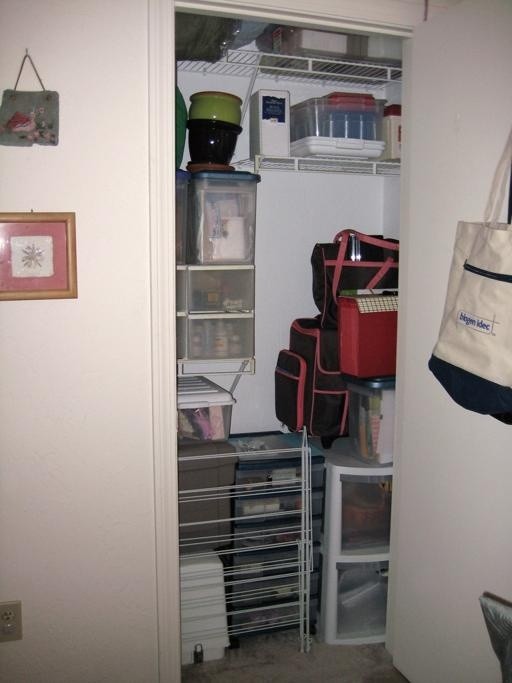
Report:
175,167,262,361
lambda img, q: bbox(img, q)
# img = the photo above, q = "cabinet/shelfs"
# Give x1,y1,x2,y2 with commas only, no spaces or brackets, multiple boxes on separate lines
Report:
175,10,401,176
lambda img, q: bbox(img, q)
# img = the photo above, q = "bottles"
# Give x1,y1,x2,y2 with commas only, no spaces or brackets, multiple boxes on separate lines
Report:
191,320,242,359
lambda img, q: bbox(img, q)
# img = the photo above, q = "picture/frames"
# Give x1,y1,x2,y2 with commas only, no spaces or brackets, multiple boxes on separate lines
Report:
1,211,79,301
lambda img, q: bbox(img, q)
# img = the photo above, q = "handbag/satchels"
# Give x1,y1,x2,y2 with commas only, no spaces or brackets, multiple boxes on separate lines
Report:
428,220,512,426
311,230,399,324
274,315,351,450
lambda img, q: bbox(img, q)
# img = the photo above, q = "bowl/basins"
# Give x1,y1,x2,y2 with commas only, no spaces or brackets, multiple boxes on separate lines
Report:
189,92,242,126
186,119,242,166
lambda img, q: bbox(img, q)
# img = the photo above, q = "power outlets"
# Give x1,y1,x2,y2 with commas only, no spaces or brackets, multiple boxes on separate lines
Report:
1,599,23,642
